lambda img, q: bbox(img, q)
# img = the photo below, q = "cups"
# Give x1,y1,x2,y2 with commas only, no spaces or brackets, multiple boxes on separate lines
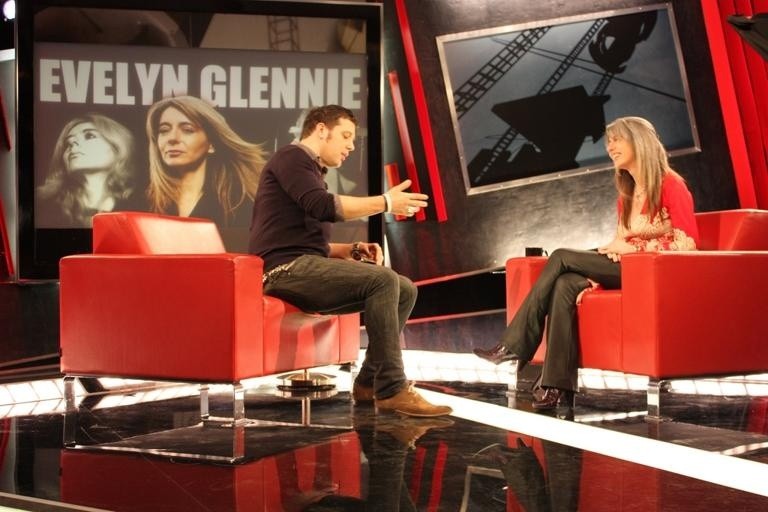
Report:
525,247,548,256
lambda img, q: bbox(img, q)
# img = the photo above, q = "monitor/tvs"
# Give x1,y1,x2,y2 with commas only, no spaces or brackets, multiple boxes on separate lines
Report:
15,0,386,284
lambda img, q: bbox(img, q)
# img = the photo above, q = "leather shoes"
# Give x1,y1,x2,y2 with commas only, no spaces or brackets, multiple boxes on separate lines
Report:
350,404,374,431
372,379,454,419
369,415,456,450
351,376,376,409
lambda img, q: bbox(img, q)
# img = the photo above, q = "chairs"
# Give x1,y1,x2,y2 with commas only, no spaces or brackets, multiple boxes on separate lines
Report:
59,211,360,465
505,209,768,424
507,431,768,512
60,431,361,512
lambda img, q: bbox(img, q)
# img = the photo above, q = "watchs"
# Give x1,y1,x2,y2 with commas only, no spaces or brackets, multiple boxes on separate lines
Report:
350,240,362,264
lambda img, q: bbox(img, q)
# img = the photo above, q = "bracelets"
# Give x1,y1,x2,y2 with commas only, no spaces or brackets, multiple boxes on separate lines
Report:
380,193,391,214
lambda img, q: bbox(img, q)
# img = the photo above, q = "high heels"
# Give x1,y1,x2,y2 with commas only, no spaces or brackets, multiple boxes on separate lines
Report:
471,341,531,374
531,386,576,412
471,437,532,465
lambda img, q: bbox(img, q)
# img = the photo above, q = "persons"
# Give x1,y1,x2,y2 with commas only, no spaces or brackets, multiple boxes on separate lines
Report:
140,97,275,227
472,436,582,512
29,111,143,229
467,116,702,412
248,104,453,420
299,409,456,512
